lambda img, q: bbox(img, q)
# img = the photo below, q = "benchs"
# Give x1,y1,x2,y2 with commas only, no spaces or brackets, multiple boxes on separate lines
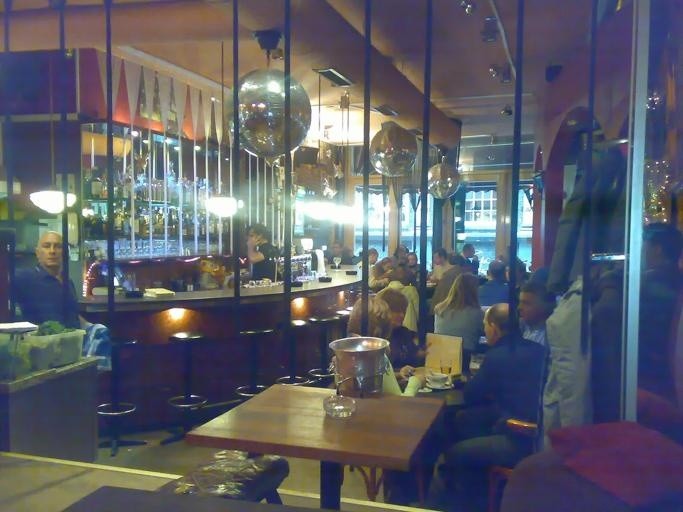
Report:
155,447,288,509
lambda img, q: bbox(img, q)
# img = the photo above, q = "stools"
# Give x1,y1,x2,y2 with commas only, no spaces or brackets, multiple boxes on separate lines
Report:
94,306,354,456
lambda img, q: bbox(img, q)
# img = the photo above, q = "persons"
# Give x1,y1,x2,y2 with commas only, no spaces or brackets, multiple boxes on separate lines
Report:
326,223,682,511
245,223,275,282
0,230,113,356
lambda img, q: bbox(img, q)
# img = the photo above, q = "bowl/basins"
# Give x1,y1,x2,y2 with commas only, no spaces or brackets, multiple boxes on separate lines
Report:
323,396,356,417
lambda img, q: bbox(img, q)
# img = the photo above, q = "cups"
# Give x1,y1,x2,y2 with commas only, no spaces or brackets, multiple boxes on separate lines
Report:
440,360,452,374
429,373,448,386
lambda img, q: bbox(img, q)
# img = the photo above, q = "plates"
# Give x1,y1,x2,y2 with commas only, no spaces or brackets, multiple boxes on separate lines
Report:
126,291,143,298
319,277,332,282
290,282,302,287
425,382,455,389
346,271,357,275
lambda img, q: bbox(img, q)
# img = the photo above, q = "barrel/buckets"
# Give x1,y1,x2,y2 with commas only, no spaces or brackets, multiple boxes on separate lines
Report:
327,336,390,400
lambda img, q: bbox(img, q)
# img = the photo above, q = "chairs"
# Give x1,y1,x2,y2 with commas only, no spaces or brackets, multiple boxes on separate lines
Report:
486,416,539,512
423,333,464,375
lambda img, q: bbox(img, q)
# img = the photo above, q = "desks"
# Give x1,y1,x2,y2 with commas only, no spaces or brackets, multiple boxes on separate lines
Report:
186,380,446,510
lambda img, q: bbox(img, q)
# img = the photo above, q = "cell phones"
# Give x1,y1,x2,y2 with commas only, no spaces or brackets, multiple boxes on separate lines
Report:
256,233,263,241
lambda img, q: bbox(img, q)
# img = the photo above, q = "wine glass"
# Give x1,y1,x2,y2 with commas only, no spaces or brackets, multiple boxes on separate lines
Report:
333,257,341,269
